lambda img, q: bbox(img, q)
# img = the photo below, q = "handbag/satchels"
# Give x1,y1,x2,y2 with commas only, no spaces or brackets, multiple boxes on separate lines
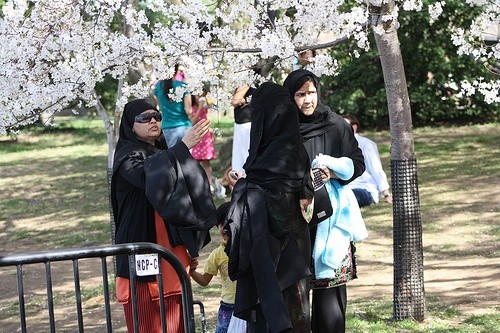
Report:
309,168,333,228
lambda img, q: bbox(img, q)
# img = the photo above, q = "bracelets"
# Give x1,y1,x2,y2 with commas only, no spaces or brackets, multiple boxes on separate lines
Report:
384,194,390,198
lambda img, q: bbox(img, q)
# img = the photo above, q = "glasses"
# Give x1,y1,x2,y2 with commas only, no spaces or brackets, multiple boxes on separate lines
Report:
134,112,162,123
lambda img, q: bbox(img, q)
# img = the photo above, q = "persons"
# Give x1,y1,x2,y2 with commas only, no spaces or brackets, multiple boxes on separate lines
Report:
109,46,392,333
